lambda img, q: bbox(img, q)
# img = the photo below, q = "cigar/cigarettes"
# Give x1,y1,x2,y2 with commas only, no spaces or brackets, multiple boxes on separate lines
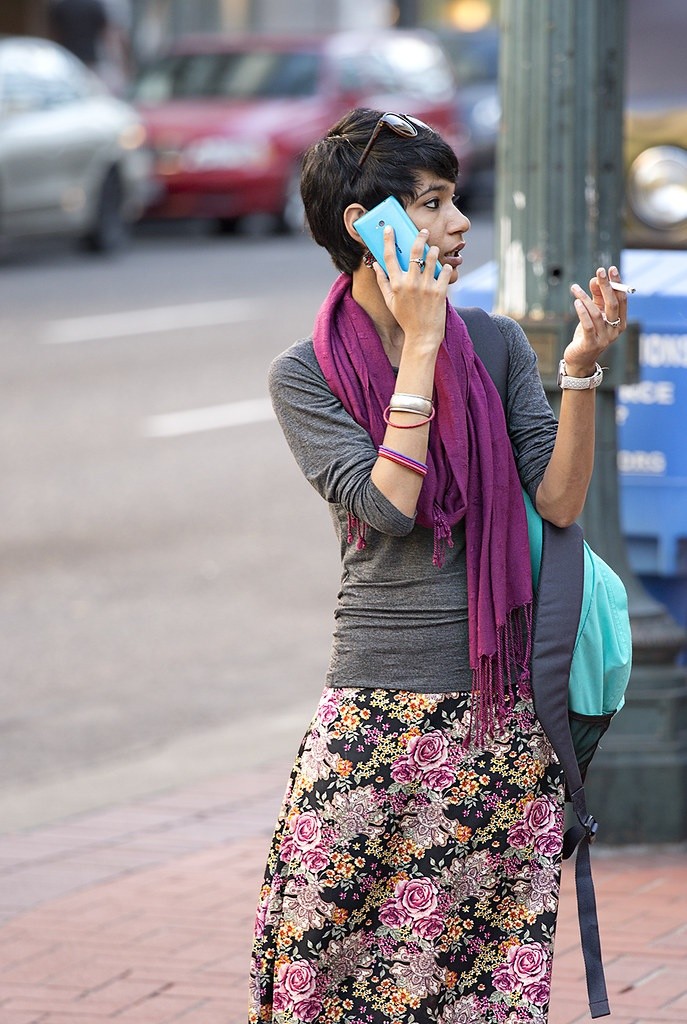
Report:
608,283,637,295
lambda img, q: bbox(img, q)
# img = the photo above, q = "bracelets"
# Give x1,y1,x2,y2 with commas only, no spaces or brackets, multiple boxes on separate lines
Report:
379,393,436,428
375,445,429,477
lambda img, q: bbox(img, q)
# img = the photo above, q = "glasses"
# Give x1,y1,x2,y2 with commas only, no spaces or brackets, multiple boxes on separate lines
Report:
348,112,436,189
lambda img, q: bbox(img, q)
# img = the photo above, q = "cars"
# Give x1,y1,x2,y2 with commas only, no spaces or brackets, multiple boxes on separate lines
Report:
451,21,501,217
0,34,151,259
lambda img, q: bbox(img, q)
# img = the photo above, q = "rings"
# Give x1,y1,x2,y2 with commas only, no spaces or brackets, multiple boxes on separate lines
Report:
603,315,622,328
410,257,426,268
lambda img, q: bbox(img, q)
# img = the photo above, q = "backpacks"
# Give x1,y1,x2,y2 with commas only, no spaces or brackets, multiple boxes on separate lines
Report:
455,305,634,860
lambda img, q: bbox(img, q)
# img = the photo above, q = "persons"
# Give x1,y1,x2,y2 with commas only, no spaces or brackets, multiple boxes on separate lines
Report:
247,103,628,1024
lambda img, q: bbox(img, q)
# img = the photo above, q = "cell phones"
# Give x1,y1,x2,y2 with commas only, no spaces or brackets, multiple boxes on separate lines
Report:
353,195,443,281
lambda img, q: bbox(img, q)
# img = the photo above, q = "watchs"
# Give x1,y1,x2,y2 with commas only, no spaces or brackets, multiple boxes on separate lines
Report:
556,359,609,392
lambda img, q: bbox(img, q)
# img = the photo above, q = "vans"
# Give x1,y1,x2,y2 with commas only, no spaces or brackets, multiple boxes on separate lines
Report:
130,25,460,236
